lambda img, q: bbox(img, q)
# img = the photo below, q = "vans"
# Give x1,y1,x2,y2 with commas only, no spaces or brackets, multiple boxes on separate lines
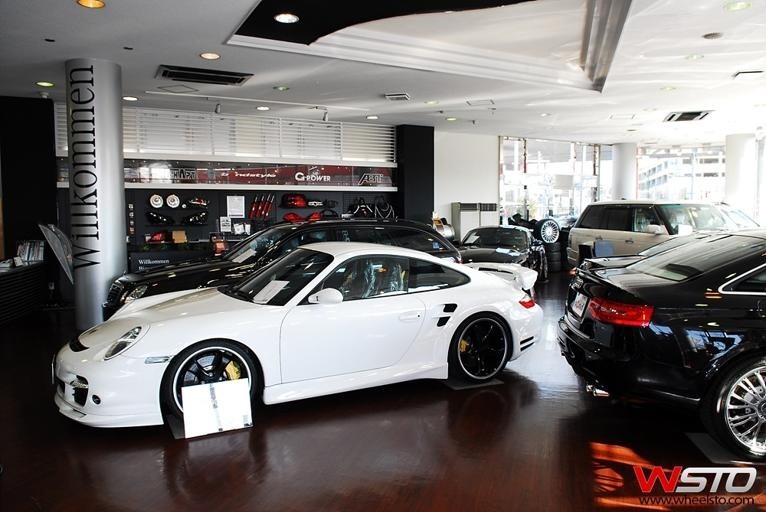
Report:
501,201,536,223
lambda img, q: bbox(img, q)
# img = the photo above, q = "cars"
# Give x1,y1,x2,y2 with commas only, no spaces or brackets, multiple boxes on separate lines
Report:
558,230,765,460
564,231,731,312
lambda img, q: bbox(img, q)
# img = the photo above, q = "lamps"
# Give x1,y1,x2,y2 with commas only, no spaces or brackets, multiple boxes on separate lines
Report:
206,96,224,117
315,105,331,124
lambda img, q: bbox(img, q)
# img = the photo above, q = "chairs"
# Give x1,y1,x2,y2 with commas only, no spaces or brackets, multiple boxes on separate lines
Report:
333,258,378,300
369,261,405,299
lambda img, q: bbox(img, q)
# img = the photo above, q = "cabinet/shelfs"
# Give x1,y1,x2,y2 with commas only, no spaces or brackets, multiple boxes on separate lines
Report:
277,201,340,222
141,201,211,246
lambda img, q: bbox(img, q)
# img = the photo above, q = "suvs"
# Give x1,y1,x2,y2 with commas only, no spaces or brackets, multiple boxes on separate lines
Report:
103,218,464,323
565,200,759,270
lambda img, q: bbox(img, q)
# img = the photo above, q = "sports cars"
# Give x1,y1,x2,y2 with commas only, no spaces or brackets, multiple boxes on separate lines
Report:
457,225,548,281
50,241,544,437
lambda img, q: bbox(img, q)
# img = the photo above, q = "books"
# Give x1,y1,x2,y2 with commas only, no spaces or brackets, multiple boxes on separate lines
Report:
16,239,45,262
